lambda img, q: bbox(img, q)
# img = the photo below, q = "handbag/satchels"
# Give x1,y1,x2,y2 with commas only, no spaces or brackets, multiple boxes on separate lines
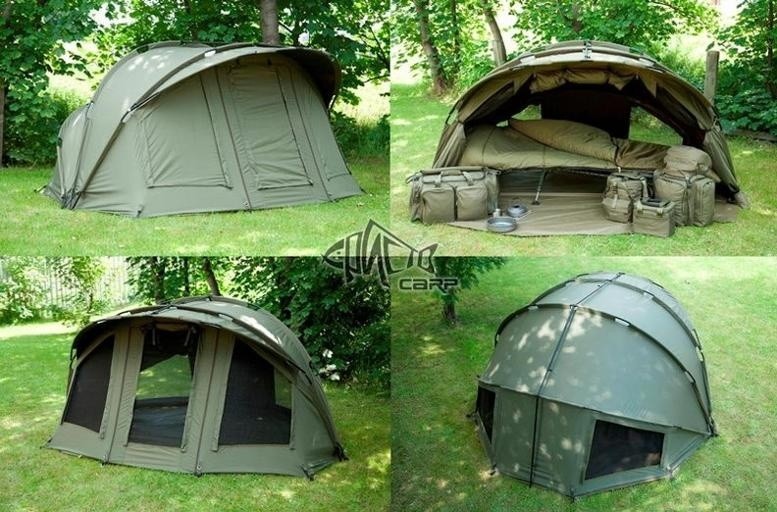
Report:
406,166,501,224
654,144,721,226
633,197,676,236
602,167,649,222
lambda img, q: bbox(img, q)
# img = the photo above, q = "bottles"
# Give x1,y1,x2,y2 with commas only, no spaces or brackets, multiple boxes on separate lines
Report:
493,209,500,217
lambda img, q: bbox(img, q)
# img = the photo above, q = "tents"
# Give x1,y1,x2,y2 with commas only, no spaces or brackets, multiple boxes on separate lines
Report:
34,39,367,218
40,294,348,482
473,271,718,501
429,39,746,235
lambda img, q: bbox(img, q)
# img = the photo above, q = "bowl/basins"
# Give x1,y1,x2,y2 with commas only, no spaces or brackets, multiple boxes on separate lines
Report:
487,218,517,233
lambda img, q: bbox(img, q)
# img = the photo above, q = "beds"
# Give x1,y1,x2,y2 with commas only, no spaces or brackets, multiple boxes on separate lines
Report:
459,125,673,204
